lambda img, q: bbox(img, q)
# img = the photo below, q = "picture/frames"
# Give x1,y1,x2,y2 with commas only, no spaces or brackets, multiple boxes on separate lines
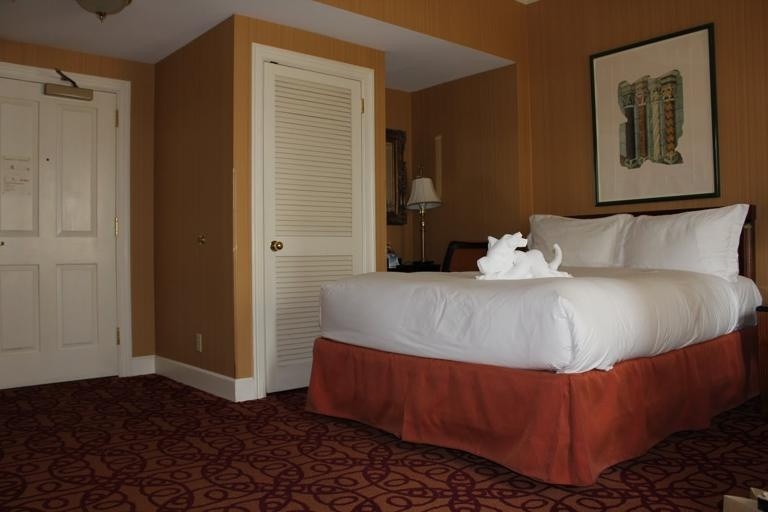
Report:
589,21,722,207
386,127,410,225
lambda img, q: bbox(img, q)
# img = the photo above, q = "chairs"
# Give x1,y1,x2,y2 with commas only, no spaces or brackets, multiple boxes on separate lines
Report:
441,242,489,272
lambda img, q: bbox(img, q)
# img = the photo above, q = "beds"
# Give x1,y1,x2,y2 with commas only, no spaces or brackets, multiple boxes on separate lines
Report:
303,204,757,488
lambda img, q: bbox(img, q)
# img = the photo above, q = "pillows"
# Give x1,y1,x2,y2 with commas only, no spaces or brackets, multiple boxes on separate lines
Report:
528,202,751,282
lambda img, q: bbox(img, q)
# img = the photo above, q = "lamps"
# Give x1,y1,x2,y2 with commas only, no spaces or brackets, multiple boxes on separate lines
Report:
406,177,442,263
76,0,132,22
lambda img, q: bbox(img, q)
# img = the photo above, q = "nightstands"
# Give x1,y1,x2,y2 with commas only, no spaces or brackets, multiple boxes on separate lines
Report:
396,264,441,272
